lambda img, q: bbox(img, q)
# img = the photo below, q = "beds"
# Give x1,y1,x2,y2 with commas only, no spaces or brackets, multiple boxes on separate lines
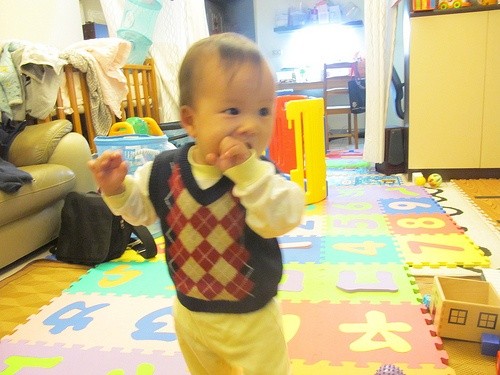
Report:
37,57,161,153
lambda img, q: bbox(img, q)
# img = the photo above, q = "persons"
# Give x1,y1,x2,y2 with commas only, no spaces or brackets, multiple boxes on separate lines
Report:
88,32,302,375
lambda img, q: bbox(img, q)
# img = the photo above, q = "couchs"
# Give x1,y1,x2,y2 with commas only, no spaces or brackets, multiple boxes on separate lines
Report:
0,119,101,270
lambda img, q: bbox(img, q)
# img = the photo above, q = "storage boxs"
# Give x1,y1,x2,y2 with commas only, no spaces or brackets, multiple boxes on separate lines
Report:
430,276,500,341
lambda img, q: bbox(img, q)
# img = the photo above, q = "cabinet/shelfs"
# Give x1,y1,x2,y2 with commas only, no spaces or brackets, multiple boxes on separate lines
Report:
409,4,500,182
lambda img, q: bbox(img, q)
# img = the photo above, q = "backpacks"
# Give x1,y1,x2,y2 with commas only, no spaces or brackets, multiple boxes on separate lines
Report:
48,191,158,264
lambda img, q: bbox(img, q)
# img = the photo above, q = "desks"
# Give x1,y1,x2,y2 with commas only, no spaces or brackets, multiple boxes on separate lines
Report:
277,76,356,92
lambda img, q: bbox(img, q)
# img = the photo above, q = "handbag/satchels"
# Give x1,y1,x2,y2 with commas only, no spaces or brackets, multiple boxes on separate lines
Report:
347,60,366,114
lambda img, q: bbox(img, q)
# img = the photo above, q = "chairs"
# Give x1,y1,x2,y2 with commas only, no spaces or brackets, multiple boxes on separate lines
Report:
324,60,358,150
